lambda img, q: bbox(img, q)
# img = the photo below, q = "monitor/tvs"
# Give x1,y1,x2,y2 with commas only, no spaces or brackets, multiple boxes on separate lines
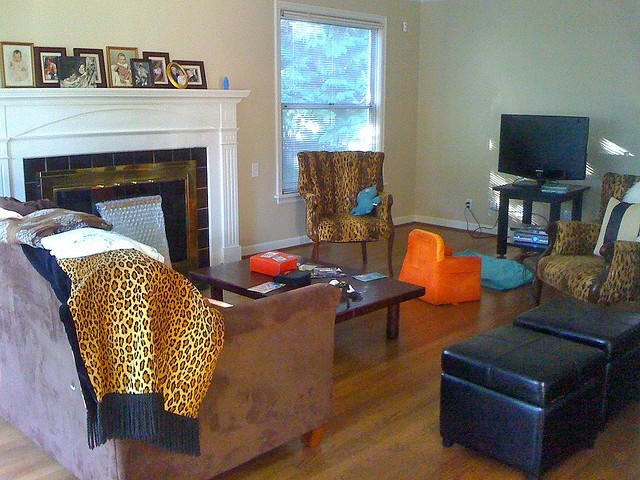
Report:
497,114,590,191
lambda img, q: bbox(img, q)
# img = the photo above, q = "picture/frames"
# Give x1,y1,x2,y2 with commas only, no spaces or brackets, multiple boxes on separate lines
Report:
106,46,141,88
131,58,157,88
172,60,208,89
33,46,68,88
141,51,172,89
72,48,108,88
57,56,98,89
0,38,36,87
167,61,189,88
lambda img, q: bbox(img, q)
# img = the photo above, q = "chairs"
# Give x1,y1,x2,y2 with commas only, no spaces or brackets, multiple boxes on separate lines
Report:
398,229,482,306
530,172,640,325
297,151,395,277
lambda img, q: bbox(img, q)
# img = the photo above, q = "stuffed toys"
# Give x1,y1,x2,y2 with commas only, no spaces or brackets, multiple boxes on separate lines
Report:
350,183,380,215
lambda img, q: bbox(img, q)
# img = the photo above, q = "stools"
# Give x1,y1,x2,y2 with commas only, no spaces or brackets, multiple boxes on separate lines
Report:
440,324,604,476
512,284,640,417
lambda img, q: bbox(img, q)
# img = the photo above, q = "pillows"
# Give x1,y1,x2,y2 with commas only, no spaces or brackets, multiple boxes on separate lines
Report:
453,248,535,291
593,196,640,257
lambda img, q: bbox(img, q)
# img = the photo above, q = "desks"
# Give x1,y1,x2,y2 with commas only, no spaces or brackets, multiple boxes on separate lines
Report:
492,180,591,259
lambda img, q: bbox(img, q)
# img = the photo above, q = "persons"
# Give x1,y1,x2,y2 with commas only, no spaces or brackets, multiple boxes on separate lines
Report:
114,53,132,87
170,67,180,85
186,69,200,83
136,63,148,86
43,58,59,80
178,71,186,86
9,50,31,83
64,63,97,84
154,67,163,78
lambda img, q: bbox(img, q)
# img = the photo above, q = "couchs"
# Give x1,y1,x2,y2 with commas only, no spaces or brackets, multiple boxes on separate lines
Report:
0,197,341,476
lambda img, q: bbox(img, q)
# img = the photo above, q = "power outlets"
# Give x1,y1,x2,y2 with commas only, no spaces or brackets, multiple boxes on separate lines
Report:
464,199,473,214
491,202,497,211
252,163,259,177
